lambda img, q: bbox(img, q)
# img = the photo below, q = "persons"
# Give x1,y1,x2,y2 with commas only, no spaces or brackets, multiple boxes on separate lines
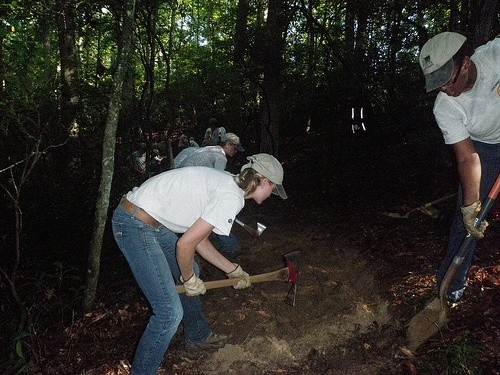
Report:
111,153,288,375
170,128,241,172
419,32,500,307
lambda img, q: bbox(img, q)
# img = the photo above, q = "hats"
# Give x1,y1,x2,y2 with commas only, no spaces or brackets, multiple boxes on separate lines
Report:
419,32,467,93
241,153,288,199
221,132,244,152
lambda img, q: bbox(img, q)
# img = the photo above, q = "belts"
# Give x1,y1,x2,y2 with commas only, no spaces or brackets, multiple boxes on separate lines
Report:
120,199,161,228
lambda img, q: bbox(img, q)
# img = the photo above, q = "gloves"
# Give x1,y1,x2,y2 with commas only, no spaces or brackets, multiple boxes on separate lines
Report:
226,263,251,290
460,200,489,238
180,273,206,297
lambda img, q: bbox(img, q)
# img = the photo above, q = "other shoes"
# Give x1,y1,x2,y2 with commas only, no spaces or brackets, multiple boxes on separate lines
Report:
425,294,459,309
187,332,227,352
231,247,249,262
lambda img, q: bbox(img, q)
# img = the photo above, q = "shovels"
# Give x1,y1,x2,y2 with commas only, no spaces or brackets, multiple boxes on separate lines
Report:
405,176,500,346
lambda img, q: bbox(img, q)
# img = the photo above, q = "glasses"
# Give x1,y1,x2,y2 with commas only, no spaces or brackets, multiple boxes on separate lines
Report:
444,62,463,87
